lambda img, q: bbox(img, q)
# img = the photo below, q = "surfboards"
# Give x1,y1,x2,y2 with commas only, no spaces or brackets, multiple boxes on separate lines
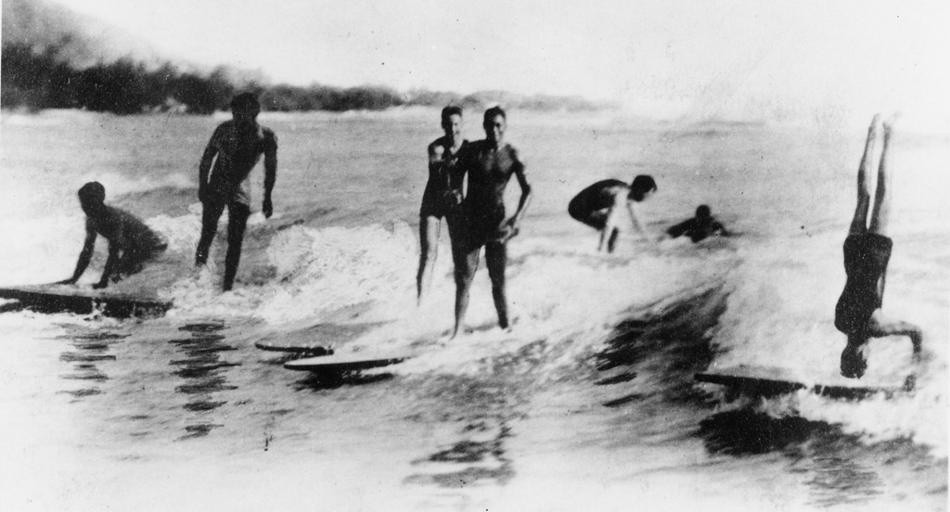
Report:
0,282,169,314
257,319,454,373
696,365,912,399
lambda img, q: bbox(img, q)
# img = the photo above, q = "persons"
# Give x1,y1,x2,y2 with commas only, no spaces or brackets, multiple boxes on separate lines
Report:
54,181,165,291
834,111,921,392
415,107,469,307
567,174,659,257
659,202,727,245
196,91,278,292
444,105,532,340
